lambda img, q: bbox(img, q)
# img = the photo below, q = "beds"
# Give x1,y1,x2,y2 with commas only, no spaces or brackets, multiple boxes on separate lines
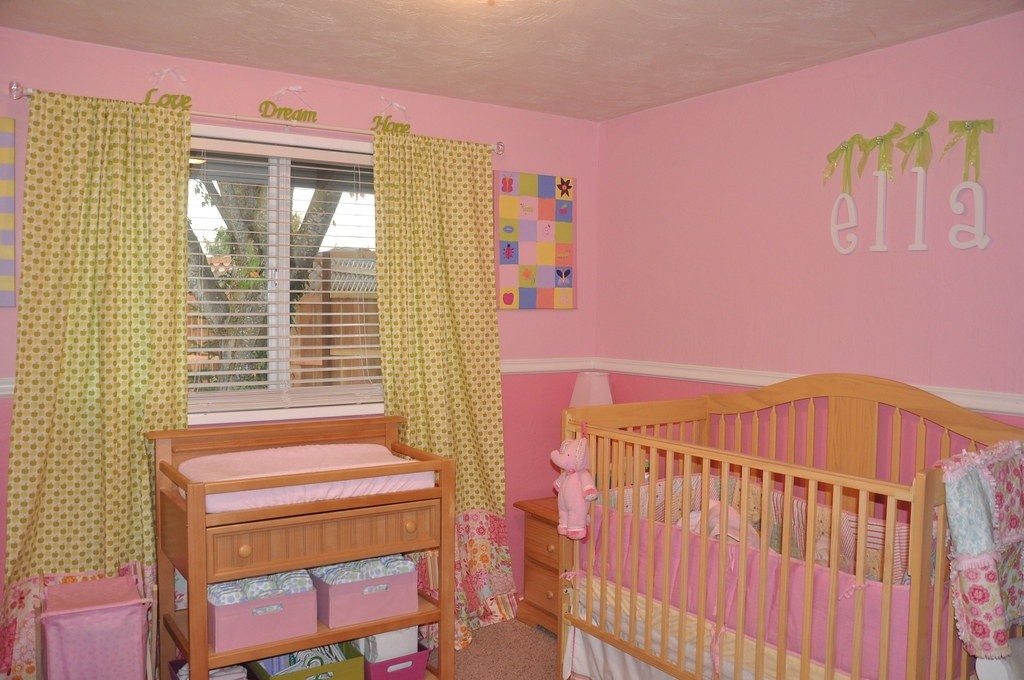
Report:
556,372,1024,680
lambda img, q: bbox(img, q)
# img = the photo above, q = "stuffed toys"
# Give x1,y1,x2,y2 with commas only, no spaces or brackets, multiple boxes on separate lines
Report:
550,436,599,540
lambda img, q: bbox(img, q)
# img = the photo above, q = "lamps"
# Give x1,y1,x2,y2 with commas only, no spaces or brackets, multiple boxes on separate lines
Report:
569,370,613,408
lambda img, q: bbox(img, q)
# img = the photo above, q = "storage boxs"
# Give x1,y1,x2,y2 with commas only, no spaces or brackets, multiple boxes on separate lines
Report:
364,641,430,680
168,659,262,680
311,569,420,628
207,588,318,653
246,643,364,680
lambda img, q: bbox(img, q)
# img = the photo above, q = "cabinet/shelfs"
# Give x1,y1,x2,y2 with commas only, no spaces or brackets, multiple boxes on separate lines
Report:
144,415,455,680
514,497,558,636
